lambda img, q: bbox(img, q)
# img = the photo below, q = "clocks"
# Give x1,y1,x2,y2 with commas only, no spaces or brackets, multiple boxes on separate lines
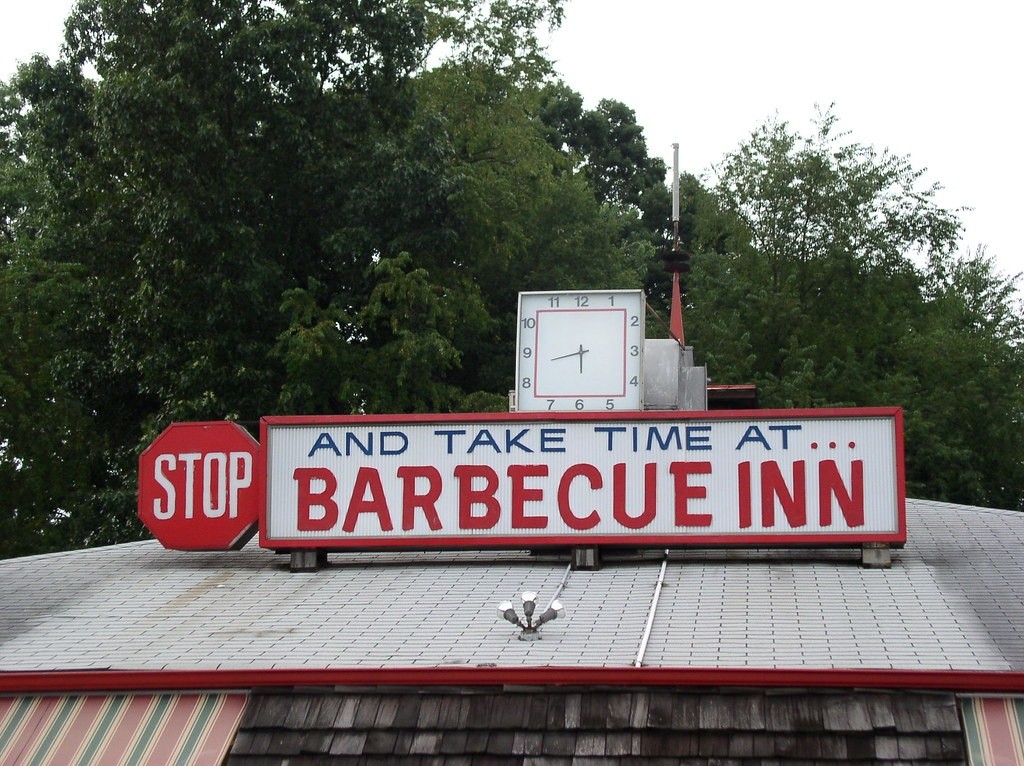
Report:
515,288,646,412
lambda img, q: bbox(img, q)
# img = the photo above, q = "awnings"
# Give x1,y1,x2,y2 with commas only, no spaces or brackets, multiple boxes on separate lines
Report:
0,688,253,766
955,693,1024,766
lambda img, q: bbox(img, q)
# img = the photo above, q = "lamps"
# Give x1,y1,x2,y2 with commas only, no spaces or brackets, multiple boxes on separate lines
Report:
496,591,565,642
